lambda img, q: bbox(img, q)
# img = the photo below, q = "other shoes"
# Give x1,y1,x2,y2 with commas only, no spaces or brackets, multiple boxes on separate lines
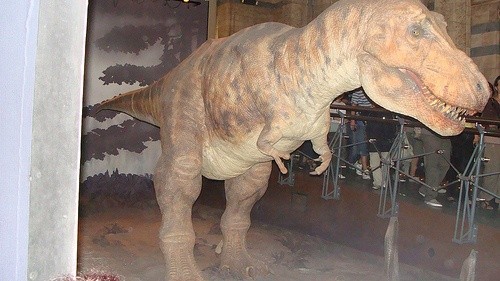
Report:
419,192,425,197
399,174,420,181
372,184,380,189
339,173,345,178
340,163,355,169
424,199,442,207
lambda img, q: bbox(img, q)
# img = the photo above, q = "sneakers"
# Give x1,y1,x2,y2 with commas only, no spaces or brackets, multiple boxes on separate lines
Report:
361,169,371,179
353,159,363,176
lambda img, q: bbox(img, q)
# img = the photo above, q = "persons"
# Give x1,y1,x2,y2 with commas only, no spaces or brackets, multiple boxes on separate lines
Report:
290,82,495,207
472,75,500,210
